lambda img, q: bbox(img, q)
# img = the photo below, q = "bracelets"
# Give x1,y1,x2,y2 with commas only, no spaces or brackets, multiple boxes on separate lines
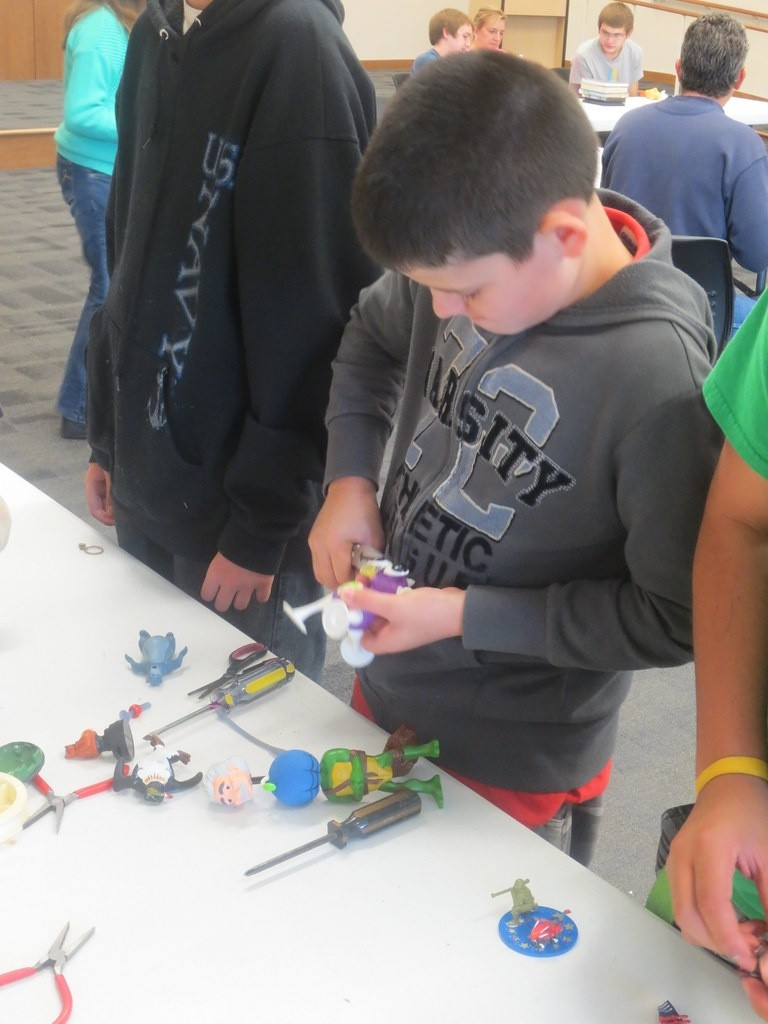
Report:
695,756,768,800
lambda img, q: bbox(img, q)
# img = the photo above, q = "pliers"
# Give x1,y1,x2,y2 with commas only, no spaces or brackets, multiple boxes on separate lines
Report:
22,764,130,833
0,922,96,1024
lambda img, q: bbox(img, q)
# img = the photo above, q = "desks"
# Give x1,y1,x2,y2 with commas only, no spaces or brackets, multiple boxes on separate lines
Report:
0,461,768,1024
577,95,768,148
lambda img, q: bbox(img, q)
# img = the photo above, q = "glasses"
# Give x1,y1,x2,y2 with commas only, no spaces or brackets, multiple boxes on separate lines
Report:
601,29,628,39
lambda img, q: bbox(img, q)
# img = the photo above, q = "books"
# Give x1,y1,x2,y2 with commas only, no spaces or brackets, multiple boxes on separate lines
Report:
579,77,629,106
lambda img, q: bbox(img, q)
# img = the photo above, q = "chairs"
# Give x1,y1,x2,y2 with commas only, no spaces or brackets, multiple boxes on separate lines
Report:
671,235,735,356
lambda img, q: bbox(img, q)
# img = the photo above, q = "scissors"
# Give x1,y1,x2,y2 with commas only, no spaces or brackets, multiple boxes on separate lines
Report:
187,643,281,699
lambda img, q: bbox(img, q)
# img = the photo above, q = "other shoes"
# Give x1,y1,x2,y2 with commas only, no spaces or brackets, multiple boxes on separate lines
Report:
61,418,90,439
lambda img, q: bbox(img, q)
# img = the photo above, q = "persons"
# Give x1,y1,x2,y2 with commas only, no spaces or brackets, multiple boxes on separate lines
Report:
307,46,726,865
602,14,768,272
568,3,644,104
411,8,475,78
54,0,385,685
664,289,768,1023
470,8,507,51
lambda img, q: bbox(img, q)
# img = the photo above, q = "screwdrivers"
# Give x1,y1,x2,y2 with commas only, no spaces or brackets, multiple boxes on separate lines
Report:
245,790,422,877
142,659,295,741
